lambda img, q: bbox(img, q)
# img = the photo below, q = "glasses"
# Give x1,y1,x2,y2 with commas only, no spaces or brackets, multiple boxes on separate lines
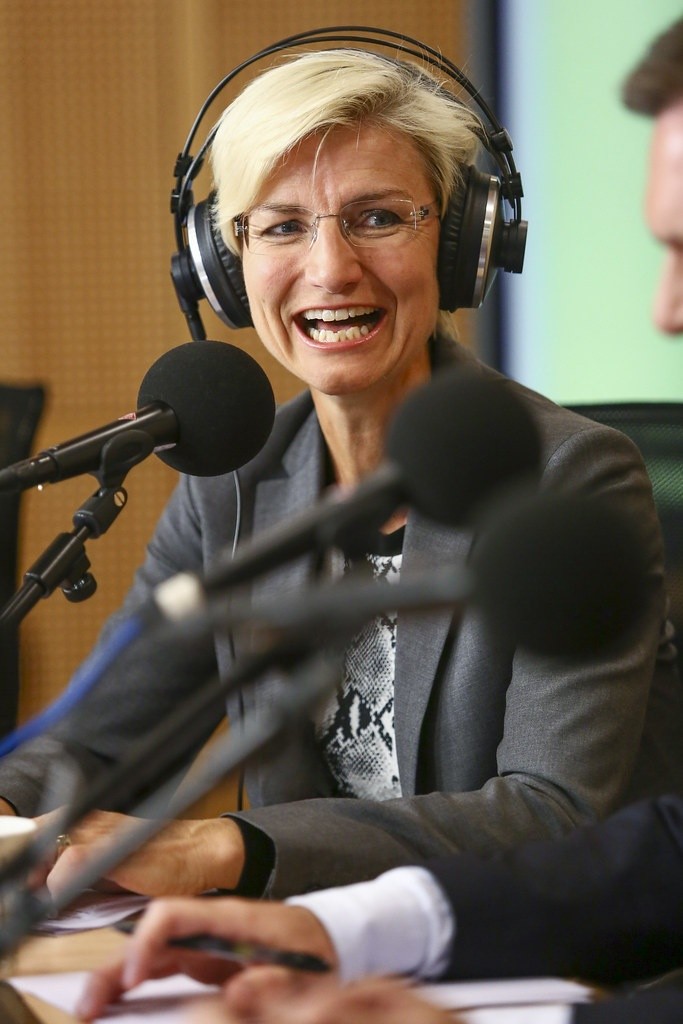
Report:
236,198,441,256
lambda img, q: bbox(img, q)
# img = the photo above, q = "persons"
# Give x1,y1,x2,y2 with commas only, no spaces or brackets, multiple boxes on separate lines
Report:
75,15,683,1023
0,28,667,899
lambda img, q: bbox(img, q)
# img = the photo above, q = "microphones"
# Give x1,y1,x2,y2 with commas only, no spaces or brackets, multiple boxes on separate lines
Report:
183,488,658,640
150,371,546,623
0,340,277,497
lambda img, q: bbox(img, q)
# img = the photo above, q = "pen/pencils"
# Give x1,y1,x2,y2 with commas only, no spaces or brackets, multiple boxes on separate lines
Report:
56,835,70,859
169,933,329,974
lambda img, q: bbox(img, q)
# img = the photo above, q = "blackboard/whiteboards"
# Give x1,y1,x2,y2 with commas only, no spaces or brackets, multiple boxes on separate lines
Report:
468,0,682,419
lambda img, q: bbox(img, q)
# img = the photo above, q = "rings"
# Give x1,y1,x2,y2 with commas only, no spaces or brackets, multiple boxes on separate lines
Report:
56,834,72,858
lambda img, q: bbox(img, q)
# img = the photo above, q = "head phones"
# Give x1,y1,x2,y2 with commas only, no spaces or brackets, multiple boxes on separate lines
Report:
169,26,527,331
